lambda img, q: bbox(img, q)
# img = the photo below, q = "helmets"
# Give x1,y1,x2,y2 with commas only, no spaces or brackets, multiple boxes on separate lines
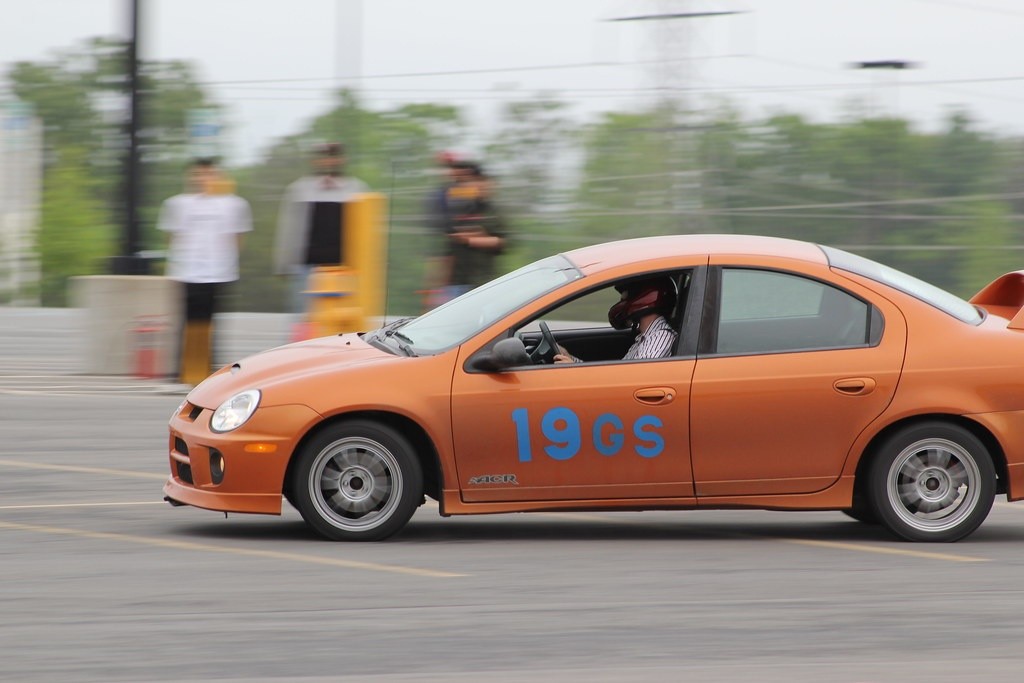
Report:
608,272,678,330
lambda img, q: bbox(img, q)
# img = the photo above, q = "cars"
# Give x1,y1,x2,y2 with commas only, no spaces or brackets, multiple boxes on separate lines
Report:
161,235,1024,545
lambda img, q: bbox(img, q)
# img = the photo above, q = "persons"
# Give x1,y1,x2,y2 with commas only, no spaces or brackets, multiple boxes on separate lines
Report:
156,154,256,325
422,148,511,314
279,140,368,282
552,273,680,364
